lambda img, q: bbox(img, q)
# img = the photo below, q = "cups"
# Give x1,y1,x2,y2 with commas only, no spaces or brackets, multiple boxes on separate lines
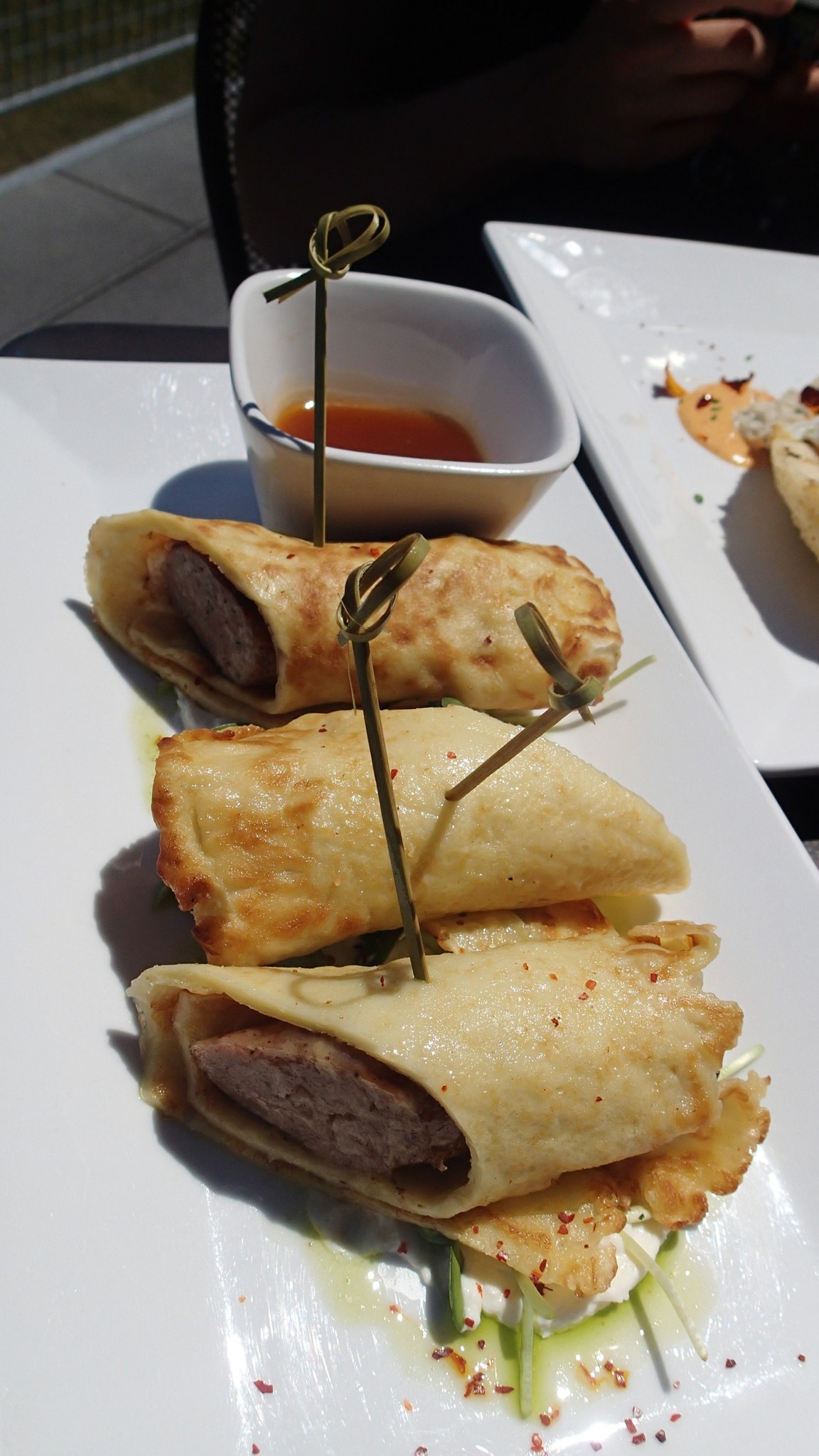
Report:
228,268,582,544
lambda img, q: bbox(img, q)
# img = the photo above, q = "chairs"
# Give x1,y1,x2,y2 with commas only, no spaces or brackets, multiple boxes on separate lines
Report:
186,0,603,321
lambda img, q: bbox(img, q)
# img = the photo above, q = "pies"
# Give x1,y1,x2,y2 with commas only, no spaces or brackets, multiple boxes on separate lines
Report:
84,507,623,726
149,707,690,969
127,922,776,1299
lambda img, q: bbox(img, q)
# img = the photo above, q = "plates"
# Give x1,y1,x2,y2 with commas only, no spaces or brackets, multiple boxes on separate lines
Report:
0,356,819,1456
483,219,819,775
